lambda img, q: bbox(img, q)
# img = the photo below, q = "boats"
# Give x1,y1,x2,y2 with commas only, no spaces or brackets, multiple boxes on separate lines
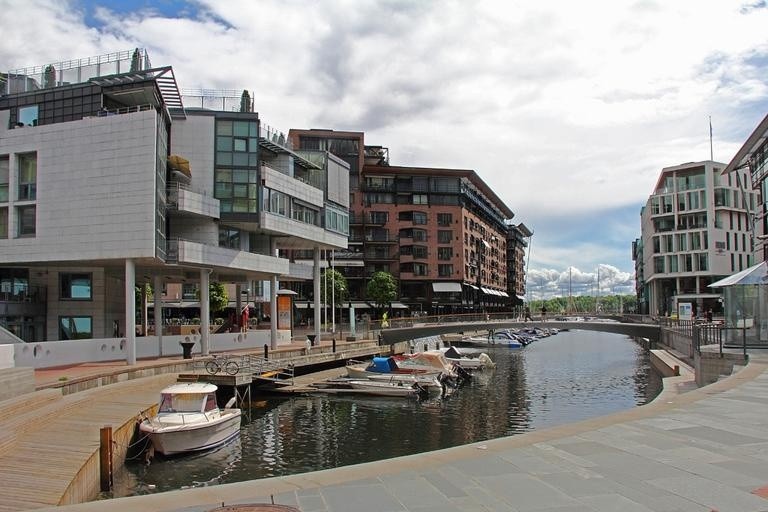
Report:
341,346,498,400
462,326,559,348
139,381,243,456
556,315,616,322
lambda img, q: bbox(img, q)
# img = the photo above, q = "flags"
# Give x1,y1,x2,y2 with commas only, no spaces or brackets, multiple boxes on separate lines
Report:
709,121,714,136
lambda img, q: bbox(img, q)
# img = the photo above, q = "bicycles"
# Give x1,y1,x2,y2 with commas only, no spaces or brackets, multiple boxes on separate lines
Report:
205,352,240,376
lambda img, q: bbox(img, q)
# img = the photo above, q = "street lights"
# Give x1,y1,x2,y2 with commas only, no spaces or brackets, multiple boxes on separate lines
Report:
339,287,344,340
246,289,250,305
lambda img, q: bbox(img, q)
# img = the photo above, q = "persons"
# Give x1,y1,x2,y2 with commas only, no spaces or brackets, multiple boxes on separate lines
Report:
707,308,713,323
524,304,534,323
486,329,496,339
540,305,548,323
